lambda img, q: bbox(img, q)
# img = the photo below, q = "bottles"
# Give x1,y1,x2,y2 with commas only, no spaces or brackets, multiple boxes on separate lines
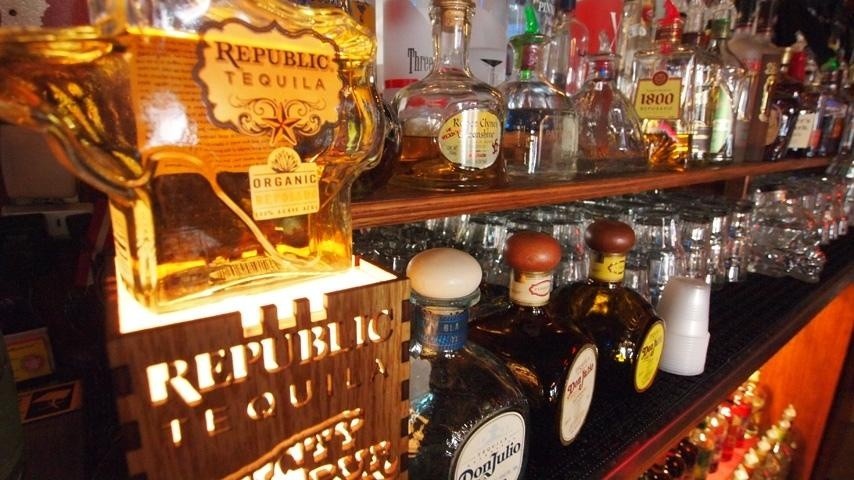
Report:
390,0,853,179
378,183,852,478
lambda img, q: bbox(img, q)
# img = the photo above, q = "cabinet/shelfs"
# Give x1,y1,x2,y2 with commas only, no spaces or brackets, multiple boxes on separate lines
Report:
352,156,854,480
